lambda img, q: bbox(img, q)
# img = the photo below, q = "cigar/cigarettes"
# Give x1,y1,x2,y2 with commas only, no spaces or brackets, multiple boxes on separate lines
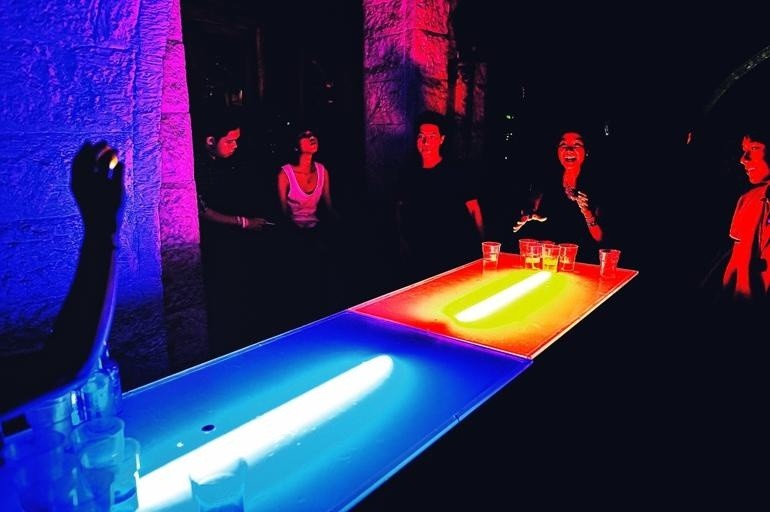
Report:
266,222,276,226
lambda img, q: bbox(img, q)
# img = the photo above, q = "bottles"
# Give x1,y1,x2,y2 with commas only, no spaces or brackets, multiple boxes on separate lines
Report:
69,344,124,423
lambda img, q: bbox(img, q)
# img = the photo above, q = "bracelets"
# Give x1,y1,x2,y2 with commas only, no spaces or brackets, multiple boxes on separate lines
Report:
237,216,247,228
585,216,598,226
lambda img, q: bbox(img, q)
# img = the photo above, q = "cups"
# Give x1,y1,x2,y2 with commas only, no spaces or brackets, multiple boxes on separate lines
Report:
597,248,621,279
185,460,251,512
2,398,140,512
600,279,615,296
518,237,578,272
482,241,502,270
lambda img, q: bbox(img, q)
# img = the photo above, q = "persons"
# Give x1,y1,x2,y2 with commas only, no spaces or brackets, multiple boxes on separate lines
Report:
386,110,483,280
512,121,613,380
721,131,770,439
191,111,276,259
0,142,123,408
274,124,332,232
